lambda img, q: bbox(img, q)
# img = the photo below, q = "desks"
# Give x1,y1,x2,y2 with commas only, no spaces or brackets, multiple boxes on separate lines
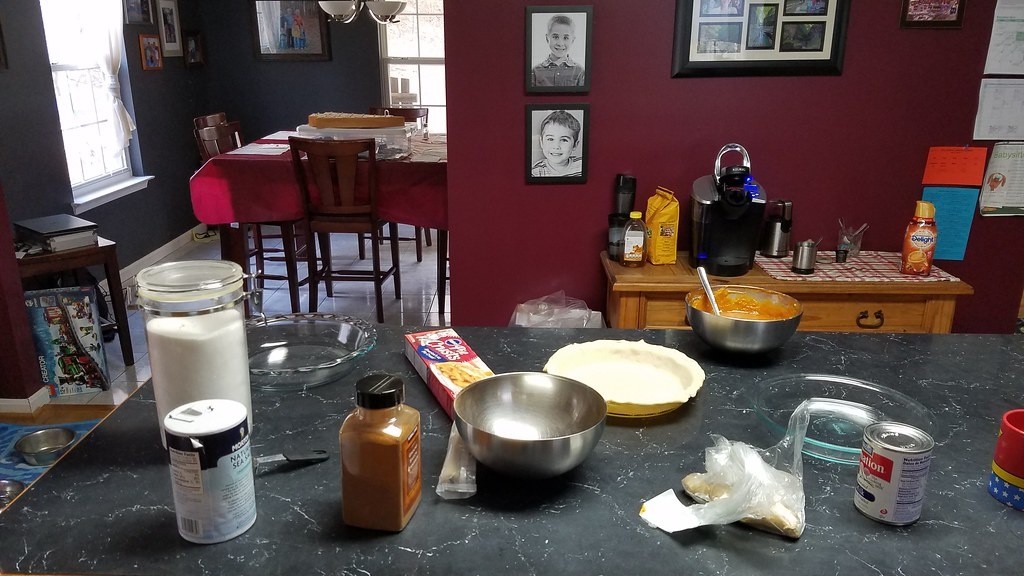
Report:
16,236,135,366
600,252,972,333
189,130,448,315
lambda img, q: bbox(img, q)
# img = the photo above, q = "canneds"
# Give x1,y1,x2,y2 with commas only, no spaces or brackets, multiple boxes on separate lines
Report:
854,418,935,526
163,399,258,546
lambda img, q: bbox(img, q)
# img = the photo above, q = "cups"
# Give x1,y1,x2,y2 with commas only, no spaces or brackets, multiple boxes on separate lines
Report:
987,409,1024,512
835,250,849,263
837,227,862,258
792,242,818,274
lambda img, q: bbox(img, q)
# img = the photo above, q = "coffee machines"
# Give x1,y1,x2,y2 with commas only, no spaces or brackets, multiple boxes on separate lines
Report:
689,143,767,277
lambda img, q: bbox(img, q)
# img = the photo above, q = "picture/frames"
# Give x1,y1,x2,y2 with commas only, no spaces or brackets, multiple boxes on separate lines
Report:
123,0,154,26
525,5,593,92
155,0,183,57
525,103,590,185
181,29,205,68
249,0,331,63
899,0,967,28
139,34,163,71
671,0,851,78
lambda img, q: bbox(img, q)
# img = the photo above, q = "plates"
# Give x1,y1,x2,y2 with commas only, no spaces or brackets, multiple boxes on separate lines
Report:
543,362,683,419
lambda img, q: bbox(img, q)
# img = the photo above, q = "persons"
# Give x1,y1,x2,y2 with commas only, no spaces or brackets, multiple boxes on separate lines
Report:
163,10,171,43
709,0,737,14
127,0,137,21
532,15,584,86
144,42,158,67
280,8,306,50
532,111,582,176
188,38,195,62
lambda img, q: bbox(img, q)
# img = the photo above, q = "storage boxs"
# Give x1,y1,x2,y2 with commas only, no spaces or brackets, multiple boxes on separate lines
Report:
24,286,111,398
405,328,496,421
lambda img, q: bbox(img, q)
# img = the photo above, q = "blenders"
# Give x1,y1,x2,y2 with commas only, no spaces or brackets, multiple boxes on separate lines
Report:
760,200,792,258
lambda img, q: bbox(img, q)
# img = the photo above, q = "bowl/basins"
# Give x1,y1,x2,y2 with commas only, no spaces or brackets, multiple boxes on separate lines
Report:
246,313,377,391
454,371,608,479
750,374,938,466
15,428,77,466
684,285,804,356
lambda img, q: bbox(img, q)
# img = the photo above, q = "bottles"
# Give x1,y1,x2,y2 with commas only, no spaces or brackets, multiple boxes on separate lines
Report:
898,201,938,277
136,260,264,450
619,211,649,268
608,213,630,262
339,374,422,532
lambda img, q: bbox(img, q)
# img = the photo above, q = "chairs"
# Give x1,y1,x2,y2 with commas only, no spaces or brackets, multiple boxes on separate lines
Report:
195,107,431,323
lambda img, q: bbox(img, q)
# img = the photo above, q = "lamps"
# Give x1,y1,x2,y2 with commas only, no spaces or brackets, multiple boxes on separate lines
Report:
318,0,407,25
